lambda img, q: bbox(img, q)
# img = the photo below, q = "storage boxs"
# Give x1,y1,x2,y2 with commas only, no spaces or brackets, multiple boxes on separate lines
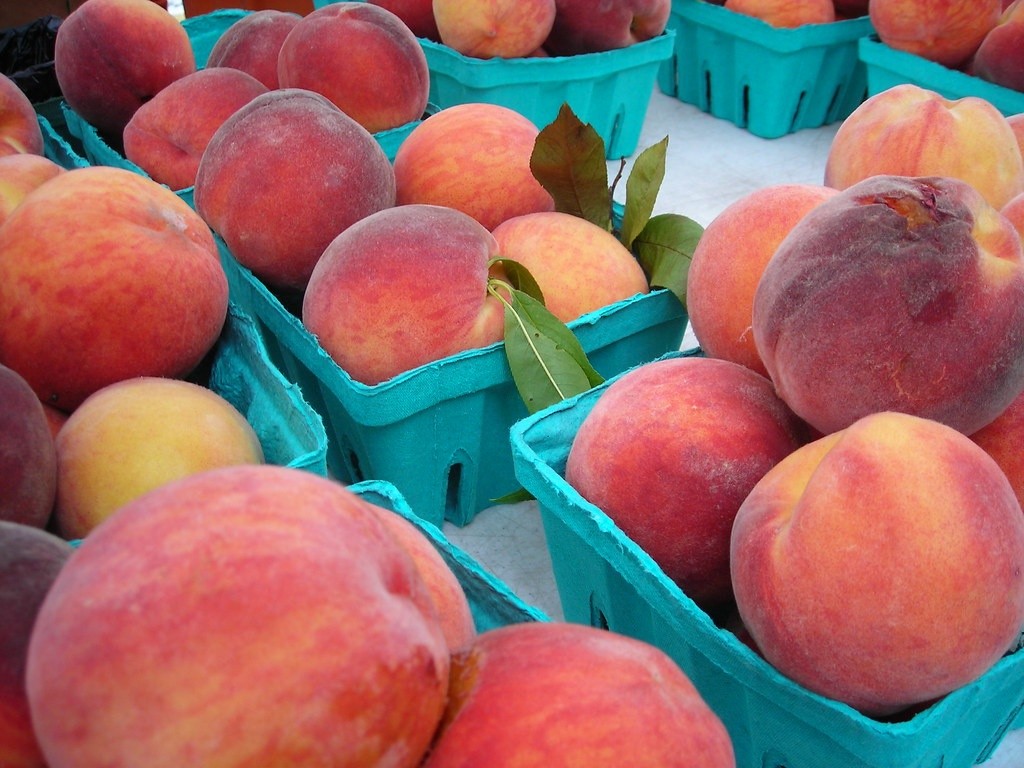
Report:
37,0,1024,768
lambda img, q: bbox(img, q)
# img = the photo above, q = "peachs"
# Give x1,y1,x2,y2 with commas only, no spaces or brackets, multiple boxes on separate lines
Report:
0,0,1023,768
